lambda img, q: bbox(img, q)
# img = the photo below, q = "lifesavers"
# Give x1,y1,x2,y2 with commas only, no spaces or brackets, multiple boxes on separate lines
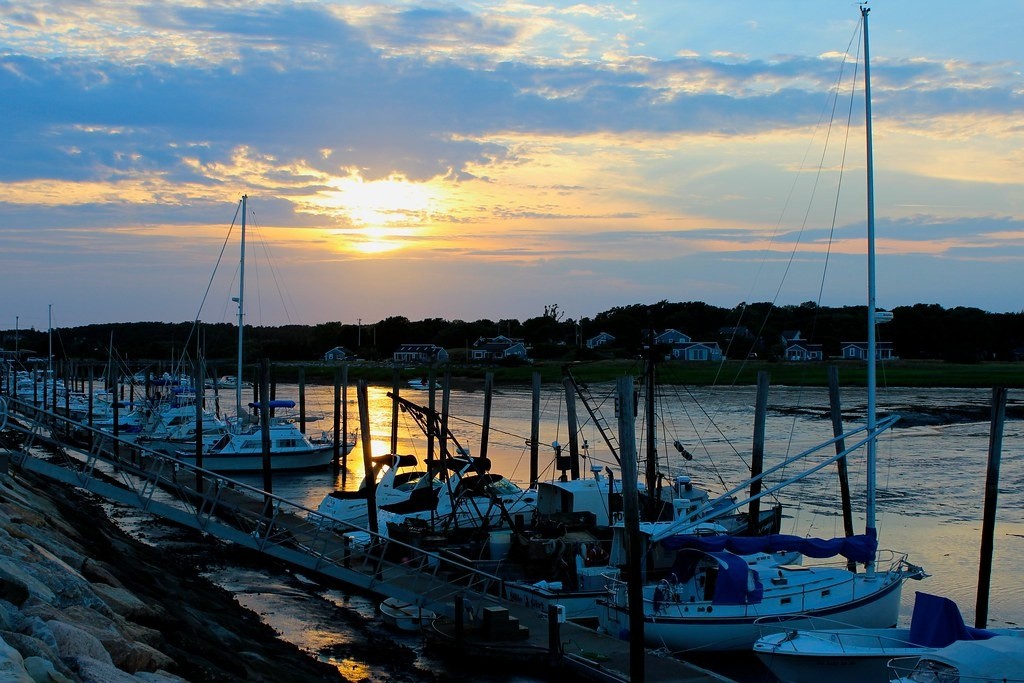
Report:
584,547,609,567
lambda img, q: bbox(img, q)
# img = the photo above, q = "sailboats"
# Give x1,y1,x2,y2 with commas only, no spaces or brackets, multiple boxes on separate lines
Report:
0,6,1024,683
